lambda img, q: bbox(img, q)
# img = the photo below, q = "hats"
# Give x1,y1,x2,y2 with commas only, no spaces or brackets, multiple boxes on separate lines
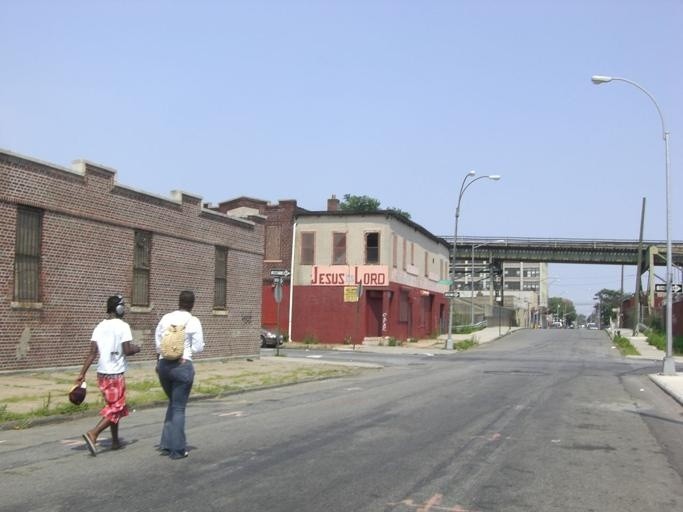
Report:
69,382,86,406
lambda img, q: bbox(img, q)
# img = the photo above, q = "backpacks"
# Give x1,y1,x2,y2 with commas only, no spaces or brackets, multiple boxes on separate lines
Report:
161,324,186,361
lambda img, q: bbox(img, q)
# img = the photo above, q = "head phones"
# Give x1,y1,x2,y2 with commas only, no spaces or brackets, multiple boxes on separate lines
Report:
115,294,124,316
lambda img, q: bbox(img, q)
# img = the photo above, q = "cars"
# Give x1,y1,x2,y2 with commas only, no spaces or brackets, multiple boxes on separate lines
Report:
261,326,283,348
588,323,598,330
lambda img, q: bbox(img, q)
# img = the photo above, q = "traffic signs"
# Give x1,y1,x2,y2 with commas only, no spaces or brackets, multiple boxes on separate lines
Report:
655,284,683,293
270,269,291,277
444,291,460,297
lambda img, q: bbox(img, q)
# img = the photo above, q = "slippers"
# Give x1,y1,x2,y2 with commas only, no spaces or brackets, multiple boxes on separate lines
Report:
82,433,97,455
112,440,126,450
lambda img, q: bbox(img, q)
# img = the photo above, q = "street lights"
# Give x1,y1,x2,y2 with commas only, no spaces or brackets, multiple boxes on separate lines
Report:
471,238,505,325
591,75,674,357
448,169,500,339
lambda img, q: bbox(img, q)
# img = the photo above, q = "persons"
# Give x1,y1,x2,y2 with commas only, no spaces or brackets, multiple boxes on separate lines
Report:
154,291,206,460
74,296,140,456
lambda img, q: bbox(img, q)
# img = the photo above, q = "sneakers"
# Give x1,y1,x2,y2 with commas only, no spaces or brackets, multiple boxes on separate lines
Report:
163,448,189,458
